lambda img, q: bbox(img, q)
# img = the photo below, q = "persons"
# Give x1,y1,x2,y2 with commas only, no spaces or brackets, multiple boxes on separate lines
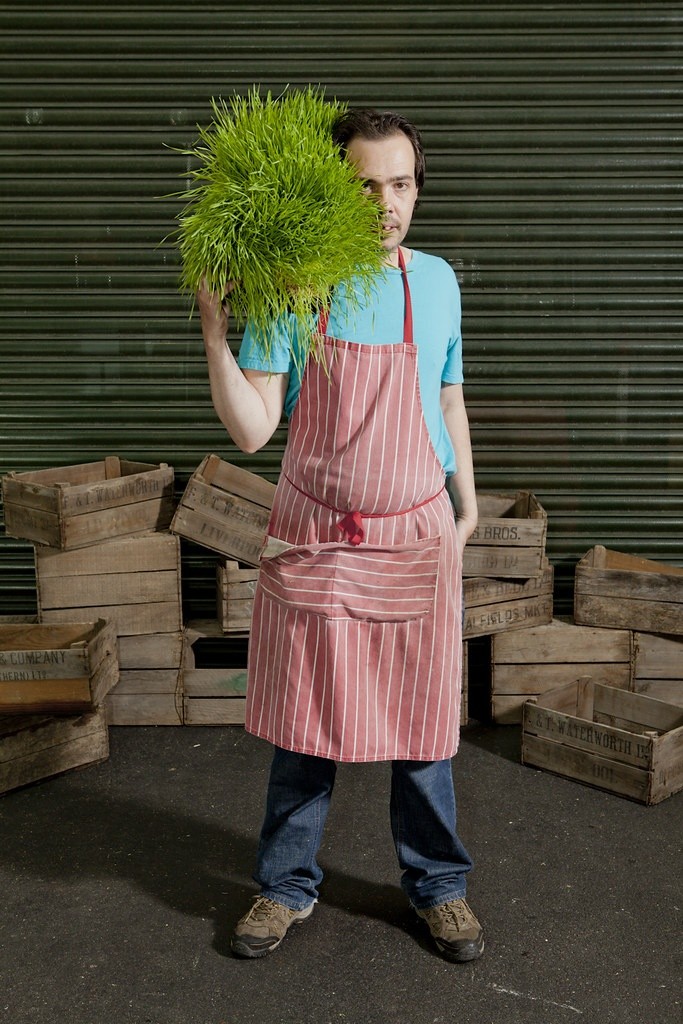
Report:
195,112,486,964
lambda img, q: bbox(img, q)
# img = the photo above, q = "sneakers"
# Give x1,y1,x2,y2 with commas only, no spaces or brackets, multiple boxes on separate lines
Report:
230,893,315,958
414,897,485,963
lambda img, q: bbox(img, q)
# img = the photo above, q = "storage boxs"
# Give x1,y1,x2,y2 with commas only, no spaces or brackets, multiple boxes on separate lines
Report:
0,453,683,807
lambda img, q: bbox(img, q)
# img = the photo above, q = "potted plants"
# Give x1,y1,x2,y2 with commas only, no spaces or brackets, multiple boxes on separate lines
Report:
156,79,413,386
